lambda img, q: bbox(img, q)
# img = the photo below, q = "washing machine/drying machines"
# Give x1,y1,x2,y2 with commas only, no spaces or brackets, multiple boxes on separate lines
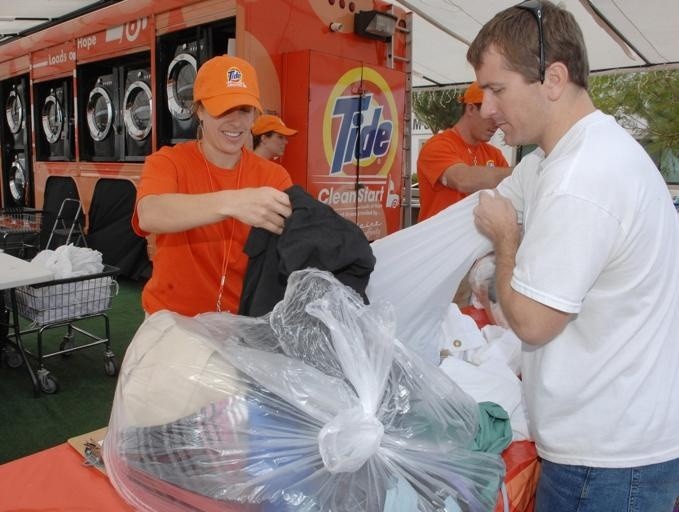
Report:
167,36,212,141
1,72,30,149
1,152,33,207
120,57,153,163
32,76,73,161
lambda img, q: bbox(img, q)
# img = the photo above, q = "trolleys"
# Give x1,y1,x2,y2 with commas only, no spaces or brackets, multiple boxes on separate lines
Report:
0,199,118,396
0,206,46,259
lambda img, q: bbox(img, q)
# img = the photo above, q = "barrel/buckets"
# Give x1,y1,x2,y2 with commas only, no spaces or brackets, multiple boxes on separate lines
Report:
78,61,120,161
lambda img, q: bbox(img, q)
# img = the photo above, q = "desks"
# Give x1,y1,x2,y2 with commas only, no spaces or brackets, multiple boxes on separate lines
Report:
1,304,543,512
0,250,55,400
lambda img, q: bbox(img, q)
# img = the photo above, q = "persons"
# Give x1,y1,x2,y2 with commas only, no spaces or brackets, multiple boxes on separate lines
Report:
417,77,520,223
248,115,297,163
465,0,679,511
127,54,294,324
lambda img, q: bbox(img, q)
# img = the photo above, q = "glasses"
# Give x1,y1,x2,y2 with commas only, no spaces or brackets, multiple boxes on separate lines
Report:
515,0,545,85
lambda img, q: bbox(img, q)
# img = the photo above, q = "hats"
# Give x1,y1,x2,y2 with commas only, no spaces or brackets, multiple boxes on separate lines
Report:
250,114,298,137
192,53,265,119
458,81,484,104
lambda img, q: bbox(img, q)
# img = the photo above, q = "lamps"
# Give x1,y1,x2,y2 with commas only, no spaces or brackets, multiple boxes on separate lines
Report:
352,9,398,44
